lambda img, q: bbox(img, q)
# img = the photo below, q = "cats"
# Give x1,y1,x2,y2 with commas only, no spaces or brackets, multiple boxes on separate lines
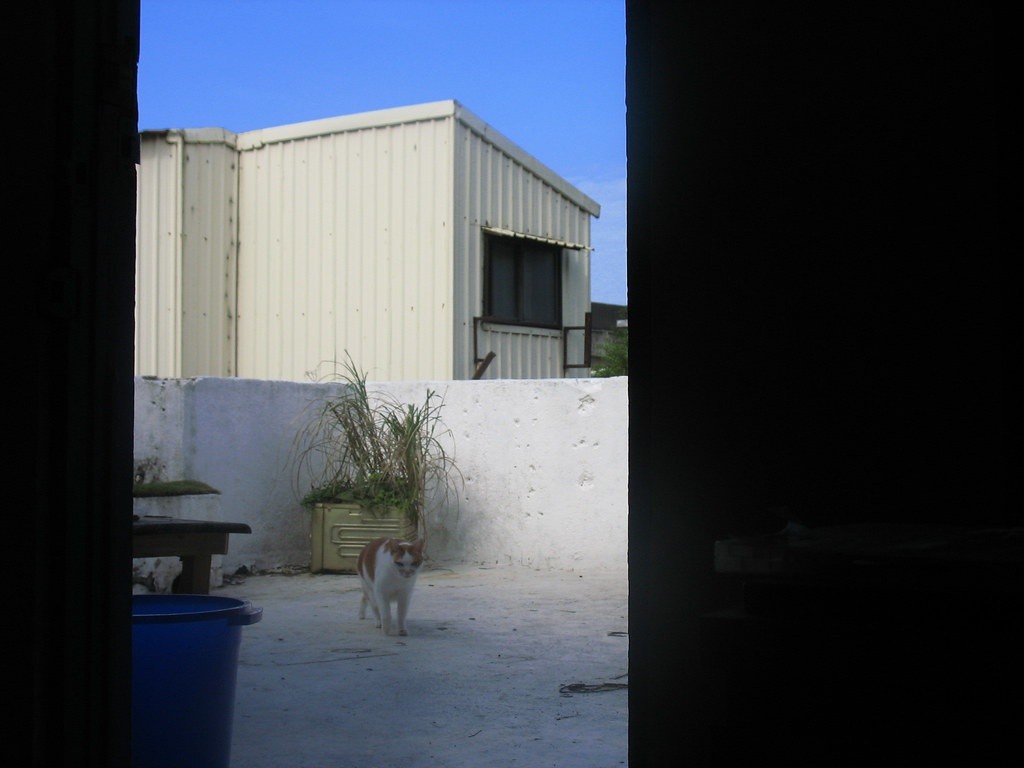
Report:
356,533,427,636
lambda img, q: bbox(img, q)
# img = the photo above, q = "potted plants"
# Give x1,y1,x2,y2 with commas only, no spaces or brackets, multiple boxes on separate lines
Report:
282,349,464,575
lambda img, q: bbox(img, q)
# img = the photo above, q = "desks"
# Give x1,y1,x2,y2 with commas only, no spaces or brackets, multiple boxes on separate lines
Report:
133,512,253,596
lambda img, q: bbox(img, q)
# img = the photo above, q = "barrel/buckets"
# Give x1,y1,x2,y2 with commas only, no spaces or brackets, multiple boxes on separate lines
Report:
133,593,264,768
